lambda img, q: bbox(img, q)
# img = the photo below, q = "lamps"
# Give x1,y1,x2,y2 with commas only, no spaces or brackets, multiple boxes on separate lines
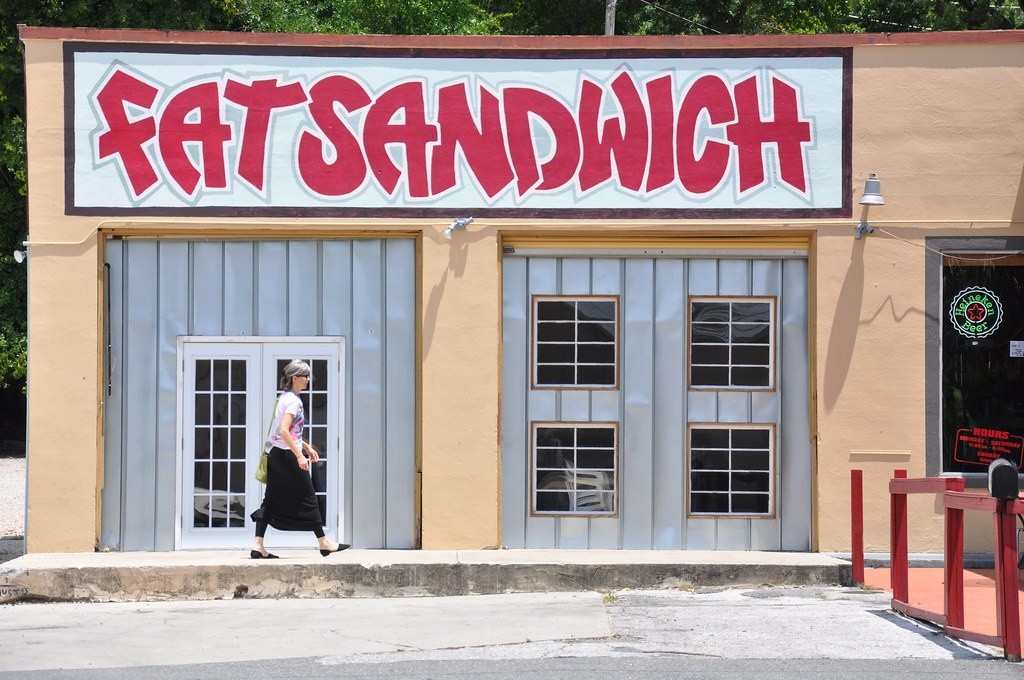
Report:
854,173,885,240
439,217,475,239
14,250,27,264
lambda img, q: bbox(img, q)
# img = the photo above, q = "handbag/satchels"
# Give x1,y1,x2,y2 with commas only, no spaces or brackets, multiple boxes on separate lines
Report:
255,451,268,484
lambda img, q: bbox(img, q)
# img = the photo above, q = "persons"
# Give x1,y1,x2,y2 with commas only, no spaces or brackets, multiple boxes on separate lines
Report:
534,428,569,513
251,360,350,560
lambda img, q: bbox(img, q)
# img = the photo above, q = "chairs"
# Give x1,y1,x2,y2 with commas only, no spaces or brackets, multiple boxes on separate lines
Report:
563,459,614,511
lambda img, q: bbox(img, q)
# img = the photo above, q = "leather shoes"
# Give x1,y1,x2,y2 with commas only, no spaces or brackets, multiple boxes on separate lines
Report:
320,544,351,557
251,550,279,560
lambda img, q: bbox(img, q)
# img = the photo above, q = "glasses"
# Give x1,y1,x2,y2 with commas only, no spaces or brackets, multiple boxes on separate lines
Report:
291,374,309,379
